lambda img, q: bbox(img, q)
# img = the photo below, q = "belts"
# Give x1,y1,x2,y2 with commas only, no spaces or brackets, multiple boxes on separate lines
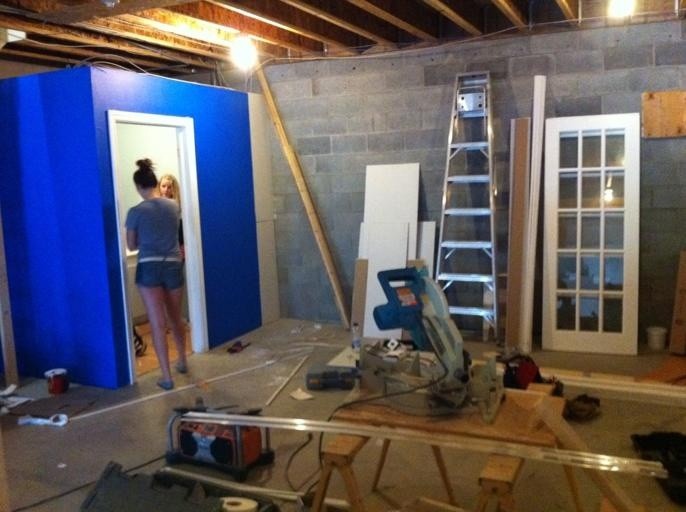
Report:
137,256,181,263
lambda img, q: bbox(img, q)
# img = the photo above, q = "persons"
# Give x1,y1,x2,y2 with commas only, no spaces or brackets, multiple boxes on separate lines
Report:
126,158,188,389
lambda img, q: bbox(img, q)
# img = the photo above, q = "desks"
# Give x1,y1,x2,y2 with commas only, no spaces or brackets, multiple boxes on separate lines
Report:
308,366,589,509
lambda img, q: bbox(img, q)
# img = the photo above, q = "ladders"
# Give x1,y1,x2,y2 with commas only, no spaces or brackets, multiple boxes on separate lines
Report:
435,69,497,342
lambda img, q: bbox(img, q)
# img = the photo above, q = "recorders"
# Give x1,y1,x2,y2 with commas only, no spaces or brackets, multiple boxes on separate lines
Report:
177,421,262,467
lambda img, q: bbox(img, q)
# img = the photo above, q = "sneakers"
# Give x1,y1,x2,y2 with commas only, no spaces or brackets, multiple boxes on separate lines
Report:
176,364,188,374
156,378,174,390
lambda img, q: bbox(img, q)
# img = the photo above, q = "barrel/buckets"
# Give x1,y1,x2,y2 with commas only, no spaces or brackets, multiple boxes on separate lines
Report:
44,367,68,395
